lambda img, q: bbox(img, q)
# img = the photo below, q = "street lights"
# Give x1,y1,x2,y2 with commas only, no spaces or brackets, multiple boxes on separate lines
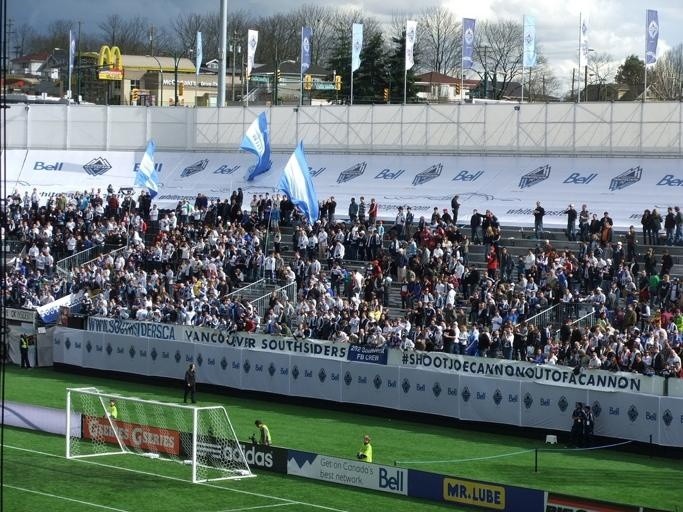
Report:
144,54,163,106
53,48,68,54
271,60,295,104
577,48,595,103
160,48,194,107
588,74,608,102
666,77,683,86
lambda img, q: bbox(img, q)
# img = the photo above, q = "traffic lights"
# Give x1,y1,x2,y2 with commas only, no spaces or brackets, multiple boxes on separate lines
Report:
132,89,139,102
455,82,461,95
383,88,389,101
179,84,184,96
276,70,280,82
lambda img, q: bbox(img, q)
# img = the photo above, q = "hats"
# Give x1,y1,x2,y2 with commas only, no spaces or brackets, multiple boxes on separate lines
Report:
255,420,261,424
111,400,116,404
364,435,370,441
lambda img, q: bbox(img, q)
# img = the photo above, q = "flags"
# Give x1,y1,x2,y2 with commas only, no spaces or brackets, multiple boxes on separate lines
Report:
238,109,270,182
299,26,313,74
275,140,317,228
69,30,76,75
645,10,660,65
404,20,418,70
195,31,202,75
579,12,589,69
461,18,475,70
132,139,159,199
246,29,259,76
351,23,363,73
522,14,536,70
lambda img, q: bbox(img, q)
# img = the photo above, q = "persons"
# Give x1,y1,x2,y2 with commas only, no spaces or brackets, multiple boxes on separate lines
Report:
255,419,271,447
102,399,117,418
355,434,373,463
1,183,683,379
563,402,584,448
182,363,197,404
582,405,593,448
